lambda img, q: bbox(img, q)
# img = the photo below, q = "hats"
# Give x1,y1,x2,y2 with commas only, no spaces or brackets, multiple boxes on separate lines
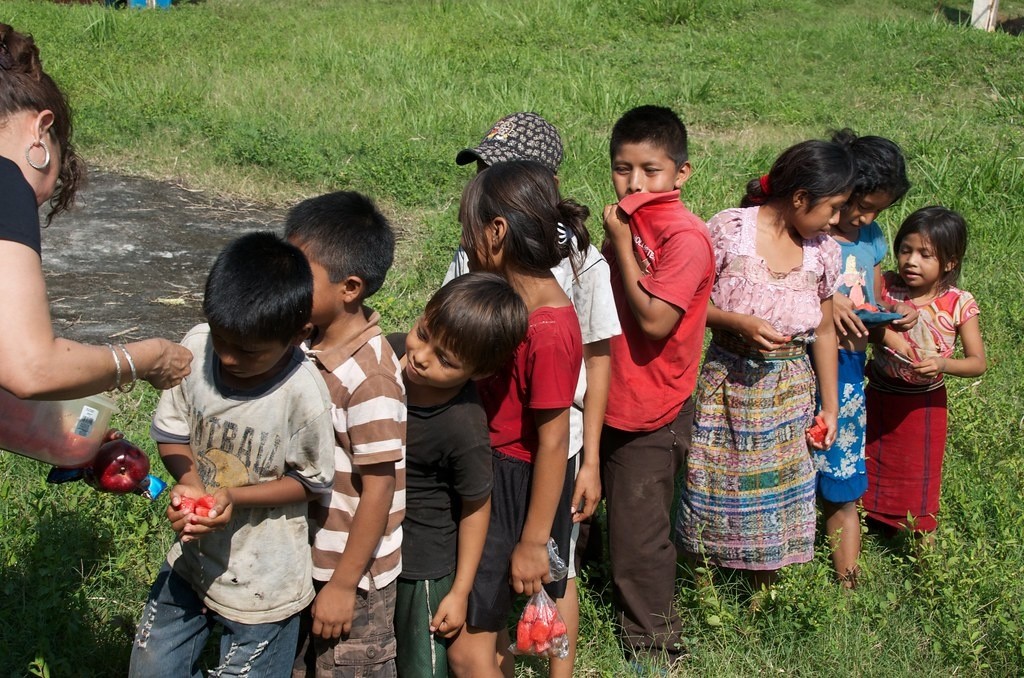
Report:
455,111,563,174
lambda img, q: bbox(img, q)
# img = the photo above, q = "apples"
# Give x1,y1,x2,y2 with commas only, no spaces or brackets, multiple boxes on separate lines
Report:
93,440,150,492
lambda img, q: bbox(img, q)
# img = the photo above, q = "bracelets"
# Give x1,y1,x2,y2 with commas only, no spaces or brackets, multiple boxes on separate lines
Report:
115,342,138,394
103,341,121,393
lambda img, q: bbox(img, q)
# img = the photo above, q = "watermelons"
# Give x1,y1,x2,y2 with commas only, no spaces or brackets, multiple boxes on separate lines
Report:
809,417,828,443
1,395,92,462
177,493,216,518
517,605,566,652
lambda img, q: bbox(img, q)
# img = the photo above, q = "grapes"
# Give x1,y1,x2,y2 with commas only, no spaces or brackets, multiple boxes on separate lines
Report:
513,637,563,657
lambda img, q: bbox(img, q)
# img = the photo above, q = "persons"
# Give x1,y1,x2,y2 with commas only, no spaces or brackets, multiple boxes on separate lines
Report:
591,104,715,657
381,268,528,678
281,190,409,678
0,21,195,399
669,137,858,604
795,127,907,597
452,160,583,678
125,229,337,678
861,203,987,570
443,111,625,678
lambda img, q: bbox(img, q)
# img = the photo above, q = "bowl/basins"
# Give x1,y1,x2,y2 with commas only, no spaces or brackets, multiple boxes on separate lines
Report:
0,386,116,469
